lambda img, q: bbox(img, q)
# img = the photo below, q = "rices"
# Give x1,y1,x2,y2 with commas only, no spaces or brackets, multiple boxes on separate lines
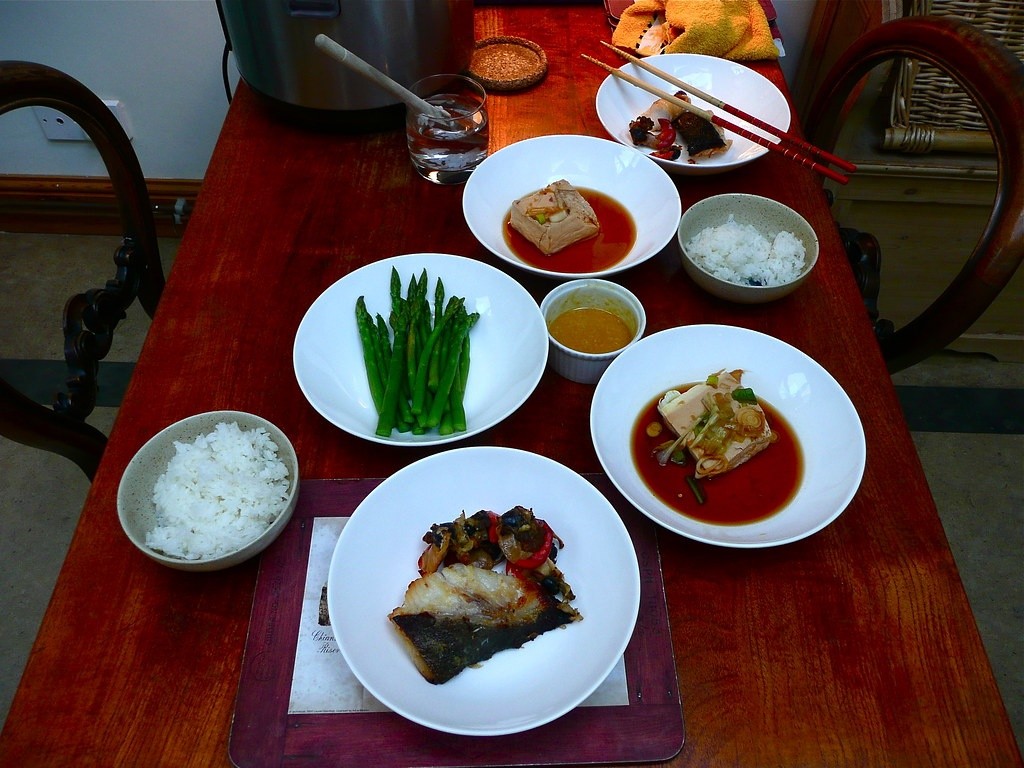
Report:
146,421,290,560
686,212,806,286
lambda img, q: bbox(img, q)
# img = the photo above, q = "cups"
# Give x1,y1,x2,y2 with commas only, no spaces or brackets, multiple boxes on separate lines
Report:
405,73,489,185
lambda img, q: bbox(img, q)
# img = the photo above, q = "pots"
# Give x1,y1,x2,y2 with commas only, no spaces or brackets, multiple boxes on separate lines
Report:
217,0,474,137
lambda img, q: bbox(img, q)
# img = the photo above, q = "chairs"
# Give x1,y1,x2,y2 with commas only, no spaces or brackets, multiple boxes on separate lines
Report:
802,15,1024,379
0,60,170,482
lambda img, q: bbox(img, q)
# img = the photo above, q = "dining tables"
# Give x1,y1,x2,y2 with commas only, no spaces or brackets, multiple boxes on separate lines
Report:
1,1,1022,768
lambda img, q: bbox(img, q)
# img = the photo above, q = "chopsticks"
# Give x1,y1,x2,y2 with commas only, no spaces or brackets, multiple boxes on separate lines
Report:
582,41,858,185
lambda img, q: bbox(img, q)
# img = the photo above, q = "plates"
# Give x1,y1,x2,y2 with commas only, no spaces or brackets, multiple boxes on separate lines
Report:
327,446,641,737
471,34,545,93
293,253,549,446
590,324,867,549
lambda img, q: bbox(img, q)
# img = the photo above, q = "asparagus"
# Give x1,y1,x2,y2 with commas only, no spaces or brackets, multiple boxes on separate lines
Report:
356,266,480,437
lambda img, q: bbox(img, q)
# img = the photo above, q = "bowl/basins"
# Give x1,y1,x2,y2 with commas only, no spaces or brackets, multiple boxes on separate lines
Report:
678,194,820,303
116,411,300,574
462,134,683,277
595,53,791,177
541,278,647,384
